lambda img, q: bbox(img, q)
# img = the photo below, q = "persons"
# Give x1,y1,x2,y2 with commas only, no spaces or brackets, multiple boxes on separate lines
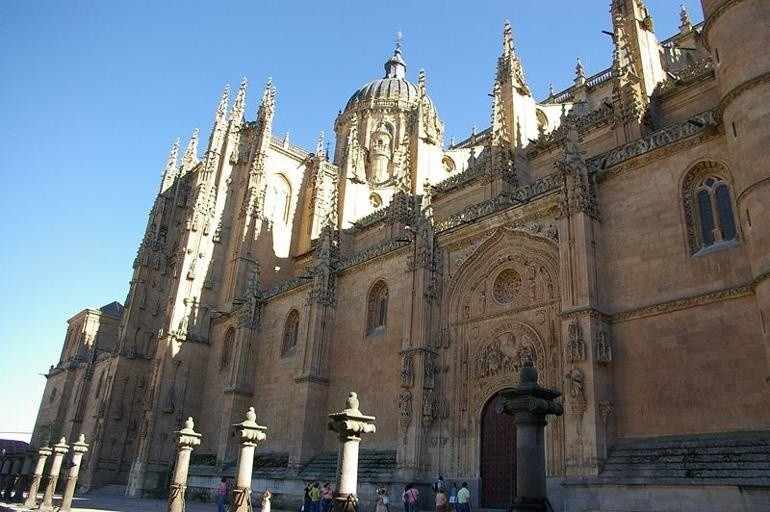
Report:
373,474,473,511
259,490,272,512
302,481,334,512
215,476,228,511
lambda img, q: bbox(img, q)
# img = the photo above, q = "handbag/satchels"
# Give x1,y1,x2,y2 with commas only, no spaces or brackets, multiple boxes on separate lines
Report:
383,496,390,505
